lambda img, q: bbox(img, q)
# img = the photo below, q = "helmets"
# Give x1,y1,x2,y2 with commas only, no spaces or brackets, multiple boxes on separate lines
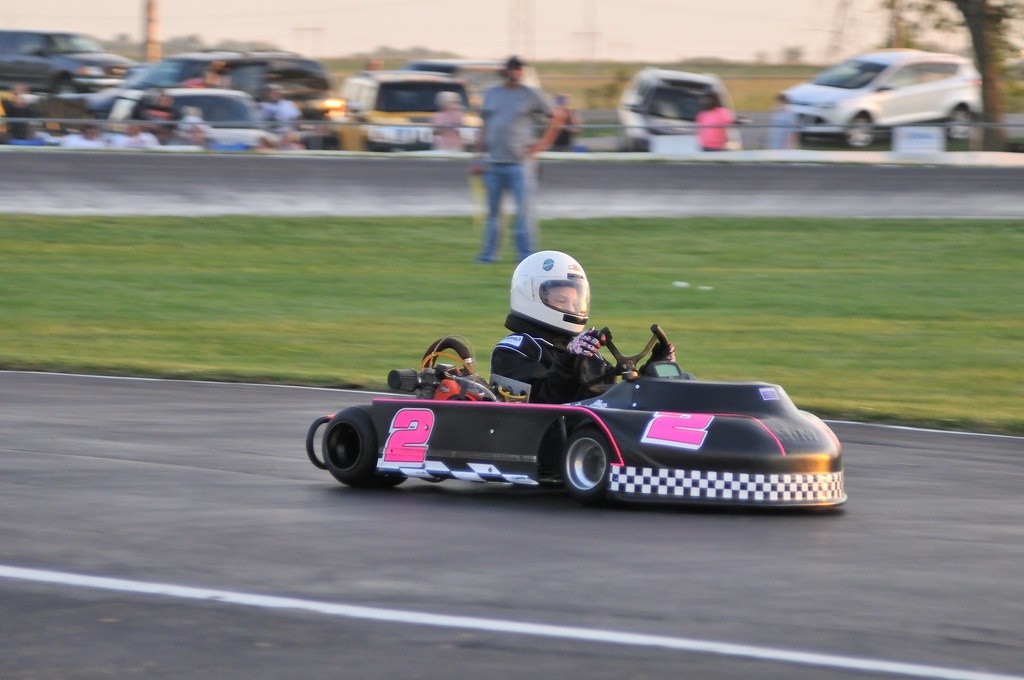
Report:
510,250,589,333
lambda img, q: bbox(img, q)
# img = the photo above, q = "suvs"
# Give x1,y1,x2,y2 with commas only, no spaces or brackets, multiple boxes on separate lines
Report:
129,46,331,102
342,69,485,153
0,29,137,94
409,60,588,148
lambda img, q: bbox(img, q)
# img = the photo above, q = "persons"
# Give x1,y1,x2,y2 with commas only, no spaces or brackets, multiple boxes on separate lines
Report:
694,93,797,152
548,94,581,151
1,58,302,150
474,56,568,264
488,251,677,406
432,91,464,153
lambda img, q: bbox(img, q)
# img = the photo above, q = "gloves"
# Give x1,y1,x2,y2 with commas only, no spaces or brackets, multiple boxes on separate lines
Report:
565,326,606,359
646,342,676,364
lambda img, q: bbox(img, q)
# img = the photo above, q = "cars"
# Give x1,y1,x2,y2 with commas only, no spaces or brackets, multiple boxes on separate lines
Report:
614,64,749,153
0,88,316,150
777,44,984,148
